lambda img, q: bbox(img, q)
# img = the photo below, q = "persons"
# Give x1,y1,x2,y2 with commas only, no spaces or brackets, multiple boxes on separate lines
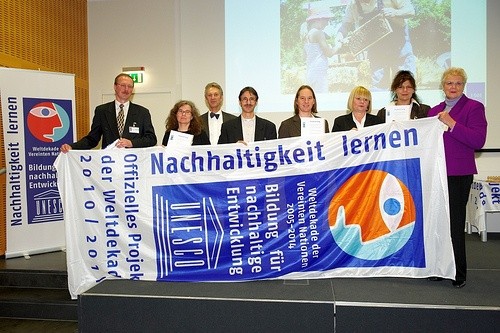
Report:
330,86,379,132
278,85,330,139
376,70,431,124
194,82,239,145
423,67,488,289
217,87,277,144
60,74,158,154
161,100,211,147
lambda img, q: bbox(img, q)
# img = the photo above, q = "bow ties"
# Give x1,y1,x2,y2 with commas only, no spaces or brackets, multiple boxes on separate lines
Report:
210,112,220,119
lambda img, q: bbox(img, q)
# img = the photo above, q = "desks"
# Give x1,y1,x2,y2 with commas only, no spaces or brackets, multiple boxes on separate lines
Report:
465,179,500,241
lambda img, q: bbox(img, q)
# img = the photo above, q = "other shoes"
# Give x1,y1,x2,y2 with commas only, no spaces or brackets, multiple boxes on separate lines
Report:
428,277,442,281
453,280,465,288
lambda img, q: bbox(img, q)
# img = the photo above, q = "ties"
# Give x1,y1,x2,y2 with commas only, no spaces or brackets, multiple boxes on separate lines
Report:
116,104,125,138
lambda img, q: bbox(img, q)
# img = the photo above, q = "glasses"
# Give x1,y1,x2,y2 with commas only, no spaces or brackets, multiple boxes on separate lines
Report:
115,83,134,89
447,82,464,86
178,110,190,114
